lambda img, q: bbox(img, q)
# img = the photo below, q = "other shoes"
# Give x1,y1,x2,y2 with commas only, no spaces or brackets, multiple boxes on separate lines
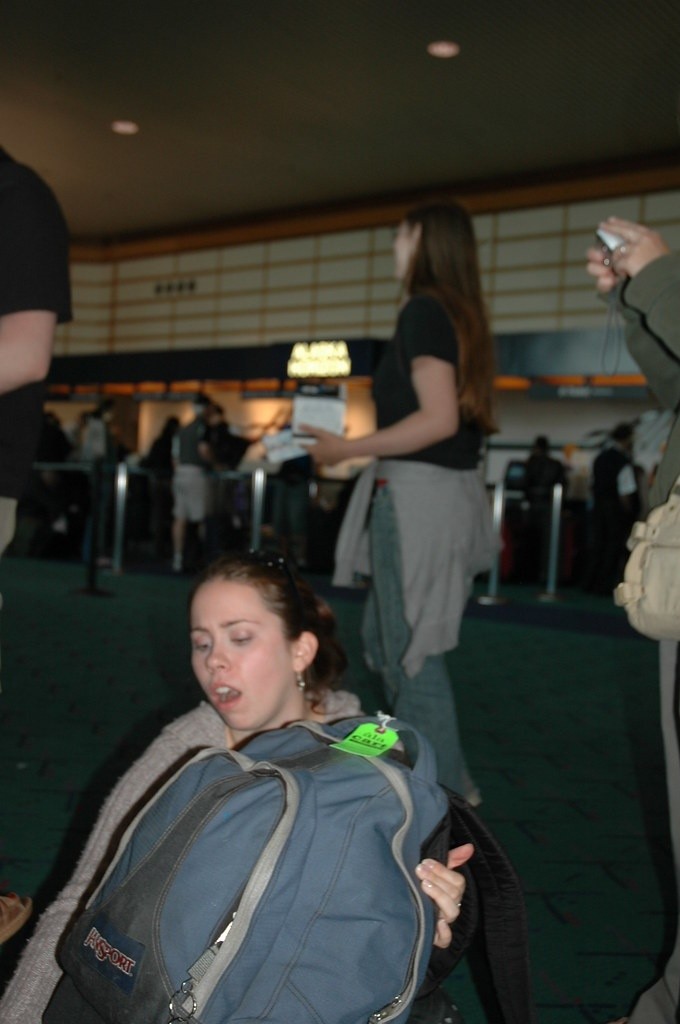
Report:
1,890,32,944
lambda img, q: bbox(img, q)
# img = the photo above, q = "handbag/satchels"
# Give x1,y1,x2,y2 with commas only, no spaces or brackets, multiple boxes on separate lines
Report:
614,478,680,642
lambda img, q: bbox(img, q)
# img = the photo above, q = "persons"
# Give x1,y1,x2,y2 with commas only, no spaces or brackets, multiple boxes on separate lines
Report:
0,146,74,549
586,216,680,1024
590,421,643,591
0,551,475,1024
30,393,316,573
299,195,493,806
521,434,564,585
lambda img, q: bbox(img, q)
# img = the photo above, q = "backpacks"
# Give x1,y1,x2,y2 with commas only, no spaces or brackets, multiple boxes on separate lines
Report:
56,710,534,1023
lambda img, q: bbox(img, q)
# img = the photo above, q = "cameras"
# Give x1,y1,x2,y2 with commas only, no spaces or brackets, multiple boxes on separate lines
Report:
595,228,625,257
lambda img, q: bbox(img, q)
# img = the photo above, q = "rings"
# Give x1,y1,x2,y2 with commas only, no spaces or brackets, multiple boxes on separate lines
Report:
457,901,461,907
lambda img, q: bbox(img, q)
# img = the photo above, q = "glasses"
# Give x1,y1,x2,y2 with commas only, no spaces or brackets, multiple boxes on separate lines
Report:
227,550,307,615
